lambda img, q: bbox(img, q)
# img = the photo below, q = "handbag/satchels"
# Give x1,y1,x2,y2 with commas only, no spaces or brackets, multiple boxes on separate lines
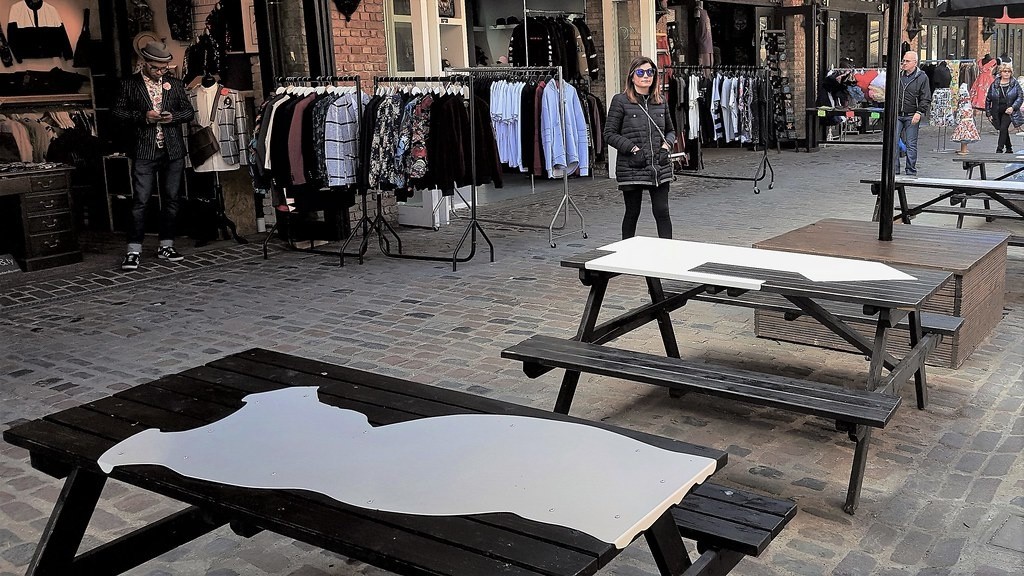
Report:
1011,109,1024,128
189,126,221,171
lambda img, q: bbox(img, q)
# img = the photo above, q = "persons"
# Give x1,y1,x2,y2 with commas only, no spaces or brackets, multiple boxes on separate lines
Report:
695,0,714,74
996,52,1013,69
950,82,982,153
968,54,996,111
184,74,249,175
985,64,1024,153
113,40,195,269
896,51,930,175
930,61,954,127
602,57,676,239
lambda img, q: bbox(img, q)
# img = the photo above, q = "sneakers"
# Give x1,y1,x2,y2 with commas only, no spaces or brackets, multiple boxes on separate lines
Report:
121,251,140,268
158,246,184,261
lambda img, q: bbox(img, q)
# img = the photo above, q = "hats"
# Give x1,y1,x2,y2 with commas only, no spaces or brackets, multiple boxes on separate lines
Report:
139,41,173,62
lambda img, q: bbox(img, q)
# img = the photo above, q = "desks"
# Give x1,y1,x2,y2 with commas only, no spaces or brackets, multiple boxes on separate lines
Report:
553,231,954,514
952,152,1024,228
2,346,730,576
860,176,1024,225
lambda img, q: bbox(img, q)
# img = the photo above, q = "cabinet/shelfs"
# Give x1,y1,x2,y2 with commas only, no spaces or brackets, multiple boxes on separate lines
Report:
102,154,188,236
0,164,84,272
0,68,99,140
388,0,431,78
427,0,470,78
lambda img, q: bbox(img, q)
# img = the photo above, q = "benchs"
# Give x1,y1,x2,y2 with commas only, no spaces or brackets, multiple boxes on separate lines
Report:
939,190,1024,202
894,204,1024,221
660,281,966,337
1007,236,1024,248
500,335,905,431
673,478,798,560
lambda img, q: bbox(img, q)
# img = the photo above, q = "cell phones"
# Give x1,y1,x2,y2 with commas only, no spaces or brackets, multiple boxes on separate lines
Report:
160,113,170,117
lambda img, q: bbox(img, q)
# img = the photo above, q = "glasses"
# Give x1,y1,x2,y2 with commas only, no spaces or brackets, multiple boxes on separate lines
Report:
1002,70,1011,73
634,68,655,77
145,61,170,74
902,59,916,62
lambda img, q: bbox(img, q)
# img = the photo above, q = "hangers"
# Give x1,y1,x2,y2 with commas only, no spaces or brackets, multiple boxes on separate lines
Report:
668,64,765,80
831,68,886,75
275,75,363,97
525,9,583,21
376,66,551,98
925,60,940,67
961,60,977,67
39,104,75,129
553,66,559,78
